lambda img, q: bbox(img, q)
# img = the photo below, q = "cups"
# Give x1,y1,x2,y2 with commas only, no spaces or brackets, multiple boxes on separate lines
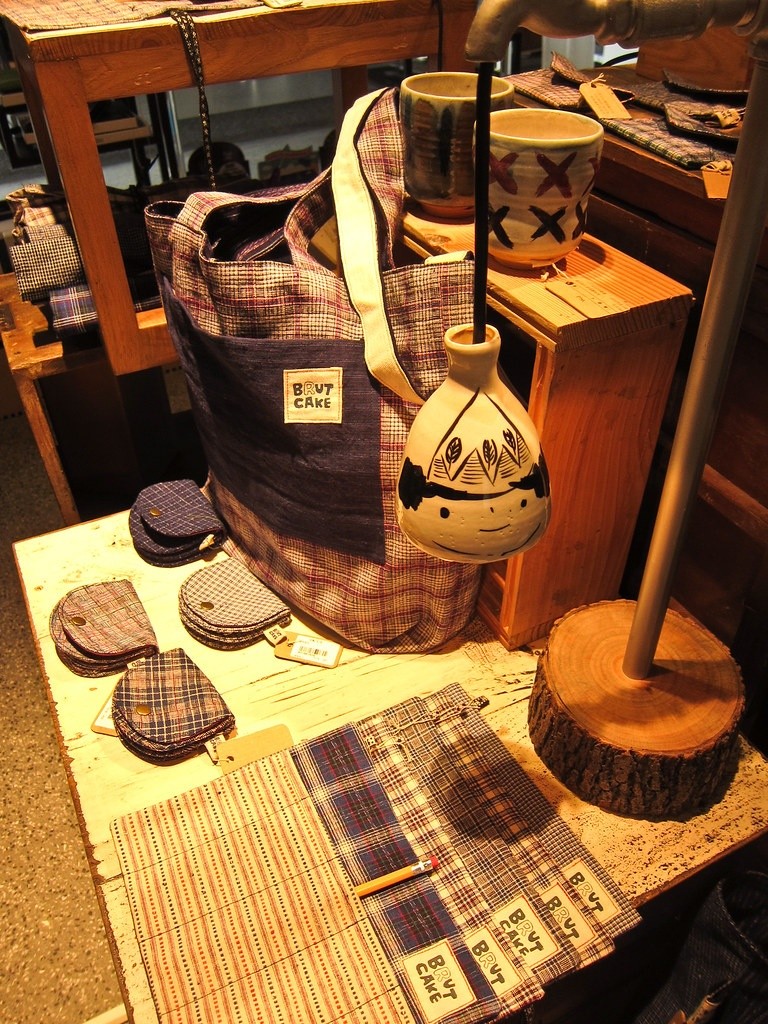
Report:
471,109,605,270
399,71,515,218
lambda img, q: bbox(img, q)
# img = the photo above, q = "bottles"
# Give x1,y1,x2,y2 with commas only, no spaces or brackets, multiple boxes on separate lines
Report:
394,320,551,561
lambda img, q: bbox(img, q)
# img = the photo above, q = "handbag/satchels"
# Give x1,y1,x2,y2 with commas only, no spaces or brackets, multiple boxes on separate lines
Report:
148,142,323,202
7,167,162,339
500,50,637,115
598,116,736,170
49,579,159,678
144,89,485,653
129,479,227,568
664,101,746,144
611,67,748,116
112,647,237,767
178,557,293,651
